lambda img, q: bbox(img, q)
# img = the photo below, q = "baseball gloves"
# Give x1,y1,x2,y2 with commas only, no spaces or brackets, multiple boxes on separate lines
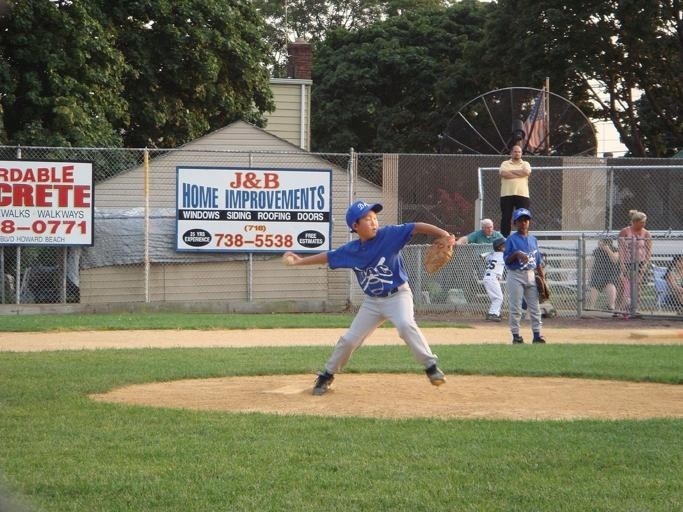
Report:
534,275,550,303
422,233,454,274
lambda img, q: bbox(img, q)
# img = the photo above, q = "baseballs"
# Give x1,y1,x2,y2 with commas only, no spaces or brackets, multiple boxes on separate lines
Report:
287,256,295,265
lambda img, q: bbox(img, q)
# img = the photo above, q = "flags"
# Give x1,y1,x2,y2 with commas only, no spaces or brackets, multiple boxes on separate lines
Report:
521,86,547,157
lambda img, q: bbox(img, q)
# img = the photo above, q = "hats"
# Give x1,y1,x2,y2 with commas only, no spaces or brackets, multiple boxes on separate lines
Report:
346,201,382,232
513,208,531,224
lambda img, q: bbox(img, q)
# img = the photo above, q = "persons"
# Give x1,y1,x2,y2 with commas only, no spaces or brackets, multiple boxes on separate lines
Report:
453,207,552,345
612,209,652,319
584,236,619,310
497,144,532,238
284,201,456,398
662,254,682,316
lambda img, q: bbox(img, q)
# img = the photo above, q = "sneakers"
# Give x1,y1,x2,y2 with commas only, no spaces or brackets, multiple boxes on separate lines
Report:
426,365,446,385
533,337,545,343
487,314,501,321
312,375,334,395
513,336,524,345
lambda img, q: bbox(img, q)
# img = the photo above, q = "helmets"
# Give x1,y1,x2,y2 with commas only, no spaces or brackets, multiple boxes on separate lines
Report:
493,238,506,252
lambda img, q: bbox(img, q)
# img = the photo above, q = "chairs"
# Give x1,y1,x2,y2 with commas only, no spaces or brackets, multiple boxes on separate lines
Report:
652,265,677,308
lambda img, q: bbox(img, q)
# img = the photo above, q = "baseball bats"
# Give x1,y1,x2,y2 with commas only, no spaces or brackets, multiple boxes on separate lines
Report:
480,251,494,259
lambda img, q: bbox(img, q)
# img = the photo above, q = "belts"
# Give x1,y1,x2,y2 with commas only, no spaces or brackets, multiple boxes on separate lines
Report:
380,288,398,297
487,273,491,276
